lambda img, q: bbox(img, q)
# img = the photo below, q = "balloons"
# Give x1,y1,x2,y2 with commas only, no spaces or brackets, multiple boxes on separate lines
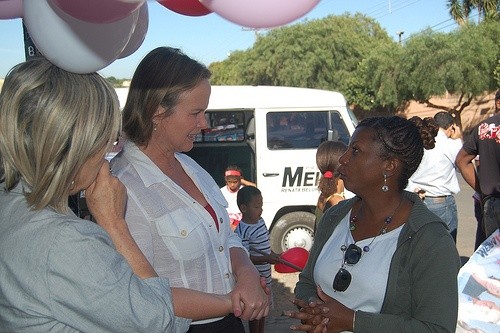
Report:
0,0,320,74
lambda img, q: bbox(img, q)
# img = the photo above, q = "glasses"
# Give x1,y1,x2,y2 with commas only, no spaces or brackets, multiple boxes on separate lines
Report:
333,244,362,292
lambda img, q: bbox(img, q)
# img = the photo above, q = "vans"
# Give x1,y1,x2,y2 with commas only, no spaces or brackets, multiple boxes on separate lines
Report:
68,86,361,259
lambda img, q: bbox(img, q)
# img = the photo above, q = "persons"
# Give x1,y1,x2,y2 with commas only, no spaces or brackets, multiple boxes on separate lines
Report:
455,114,499,239
313,140,349,237
284,116,459,333
472,90,500,251
233,185,279,333
219,165,256,232
109,47,270,333
403,111,464,245
0,58,174,333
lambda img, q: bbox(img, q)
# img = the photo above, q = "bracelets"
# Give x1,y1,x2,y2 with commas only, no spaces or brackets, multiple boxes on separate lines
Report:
352,310,357,332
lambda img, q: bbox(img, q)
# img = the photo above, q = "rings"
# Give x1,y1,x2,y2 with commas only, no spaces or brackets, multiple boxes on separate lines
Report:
109,167,113,174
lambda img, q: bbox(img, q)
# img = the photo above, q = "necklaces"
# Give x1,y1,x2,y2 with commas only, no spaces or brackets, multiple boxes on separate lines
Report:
340,195,405,266
